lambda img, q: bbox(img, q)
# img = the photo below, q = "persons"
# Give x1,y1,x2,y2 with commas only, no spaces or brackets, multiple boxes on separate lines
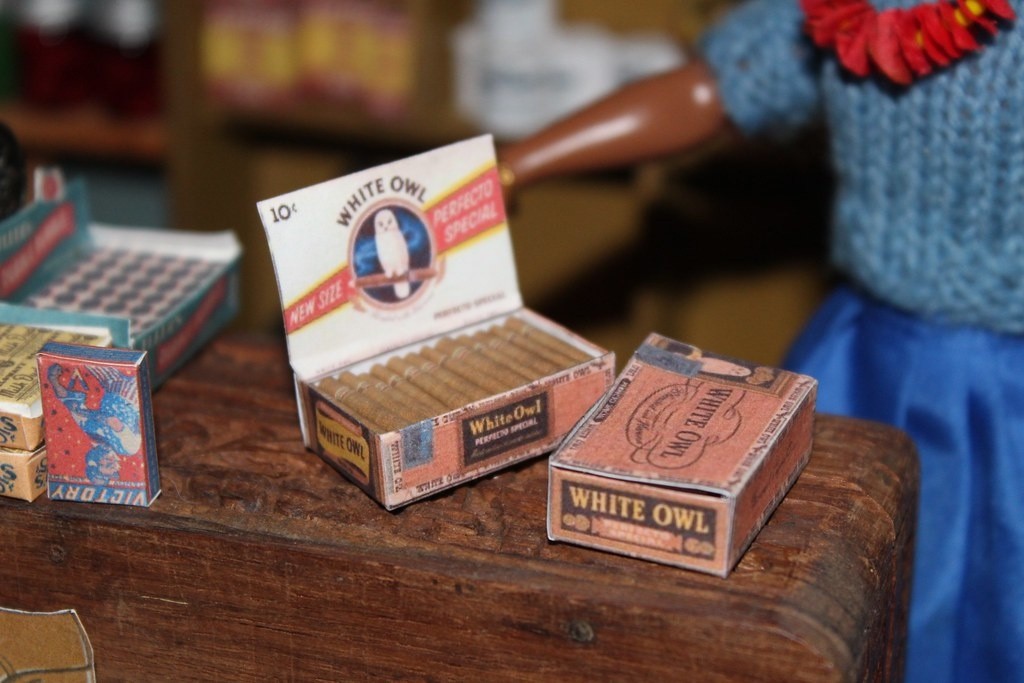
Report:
493,2,1024,683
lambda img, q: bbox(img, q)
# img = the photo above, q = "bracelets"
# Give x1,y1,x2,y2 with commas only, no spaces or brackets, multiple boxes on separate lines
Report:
496,164,520,189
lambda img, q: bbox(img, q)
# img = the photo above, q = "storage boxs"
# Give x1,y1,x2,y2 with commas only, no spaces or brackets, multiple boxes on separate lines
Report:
35,340,162,507
545,333,819,580
0,324,112,452
256,134,615,512
0,178,244,396
0,448,47,502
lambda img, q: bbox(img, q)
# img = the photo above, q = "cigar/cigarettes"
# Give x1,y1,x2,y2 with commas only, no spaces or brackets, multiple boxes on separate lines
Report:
307,314,597,436
